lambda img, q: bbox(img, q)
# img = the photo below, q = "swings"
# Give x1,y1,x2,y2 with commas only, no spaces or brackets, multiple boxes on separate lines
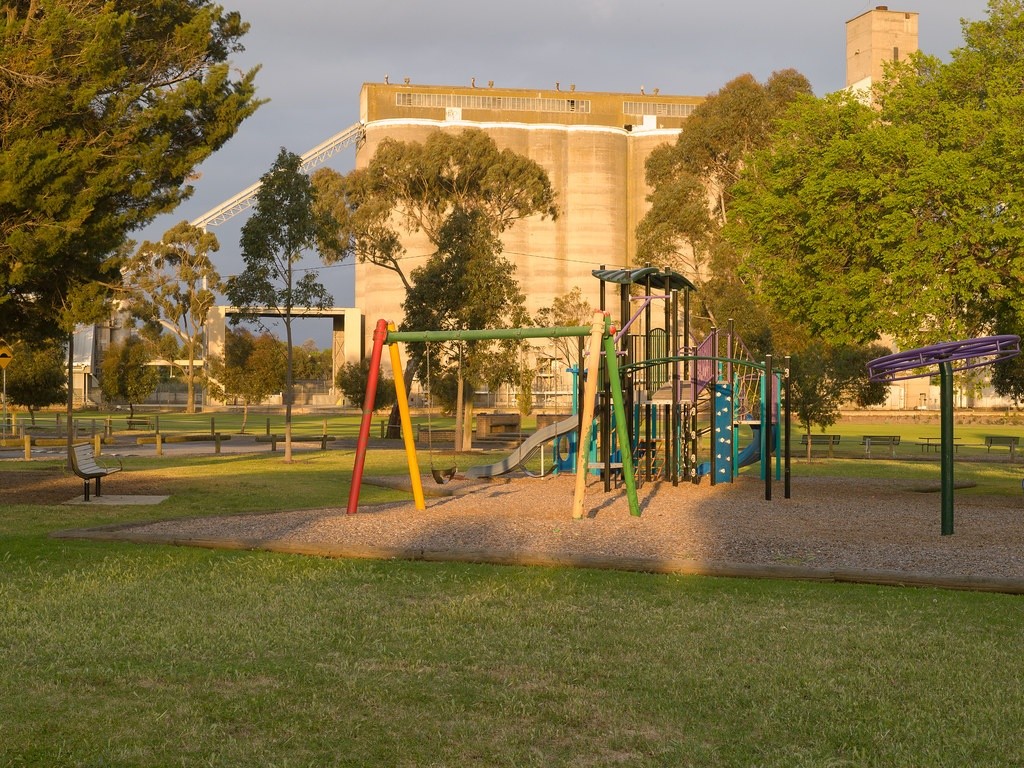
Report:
517,337,560,479
423,340,463,484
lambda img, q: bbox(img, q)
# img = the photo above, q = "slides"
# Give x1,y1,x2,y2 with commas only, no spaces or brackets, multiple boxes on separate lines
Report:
695,415,783,475
453,403,599,478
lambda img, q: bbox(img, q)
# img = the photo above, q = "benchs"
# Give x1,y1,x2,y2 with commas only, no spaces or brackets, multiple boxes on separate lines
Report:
69,442,122,502
800,434,840,459
127,417,152,430
860,436,900,459
984,435,1020,453
915,436,964,453
